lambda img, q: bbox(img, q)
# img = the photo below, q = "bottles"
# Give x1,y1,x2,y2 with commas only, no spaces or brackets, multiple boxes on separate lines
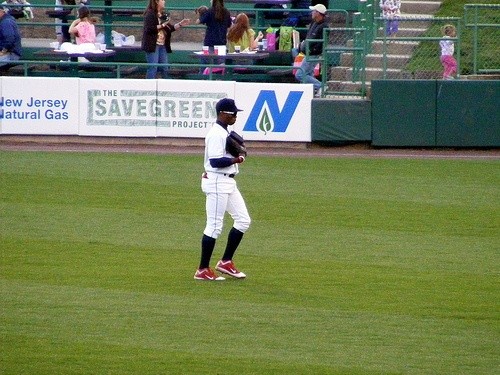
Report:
57,32,62,45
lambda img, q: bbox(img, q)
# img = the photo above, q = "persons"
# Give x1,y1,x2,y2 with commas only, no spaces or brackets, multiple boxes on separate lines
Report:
228,13,263,64
0,4,22,67
194,99,250,280
380,0,401,37
69,6,96,44
196,0,231,73
7,0,29,19
439,25,457,79
295,4,328,97
285,0,308,26
255,0,284,27
55,0,87,47
141,0,190,79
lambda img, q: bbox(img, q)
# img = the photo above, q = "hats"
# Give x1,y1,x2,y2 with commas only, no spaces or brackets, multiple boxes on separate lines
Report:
217,98,244,112
309,4,327,15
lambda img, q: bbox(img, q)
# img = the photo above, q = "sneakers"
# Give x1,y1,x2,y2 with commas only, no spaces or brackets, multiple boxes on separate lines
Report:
194,267,226,281
215,260,246,279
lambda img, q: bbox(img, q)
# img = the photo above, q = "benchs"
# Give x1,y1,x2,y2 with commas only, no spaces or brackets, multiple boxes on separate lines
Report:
90,9,145,14
45,10,71,41
268,69,293,82
186,73,273,81
10,64,36,75
245,12,331,21
31,69,117,78
114,66,138,77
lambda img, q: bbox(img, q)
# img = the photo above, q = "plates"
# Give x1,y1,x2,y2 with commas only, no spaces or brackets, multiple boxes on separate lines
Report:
54,50,67,53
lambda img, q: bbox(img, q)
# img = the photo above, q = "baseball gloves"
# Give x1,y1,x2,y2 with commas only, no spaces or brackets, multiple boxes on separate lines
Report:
226,131,247,158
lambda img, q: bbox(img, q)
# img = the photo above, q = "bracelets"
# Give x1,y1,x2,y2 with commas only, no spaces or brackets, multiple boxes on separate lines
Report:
179,23,182,27
239,156,245,161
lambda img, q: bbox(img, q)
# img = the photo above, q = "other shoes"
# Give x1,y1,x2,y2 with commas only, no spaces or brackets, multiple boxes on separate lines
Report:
315,83,328,97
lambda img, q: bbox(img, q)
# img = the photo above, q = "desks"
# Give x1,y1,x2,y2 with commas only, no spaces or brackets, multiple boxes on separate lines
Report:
34,49,115,72
191,53,270,75
107,46,141,50
257,48,291,52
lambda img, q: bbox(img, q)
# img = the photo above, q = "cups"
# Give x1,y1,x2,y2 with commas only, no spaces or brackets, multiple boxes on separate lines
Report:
257,42,264,50
100,44,106,51
95,43,101,49
234,45,241,54
